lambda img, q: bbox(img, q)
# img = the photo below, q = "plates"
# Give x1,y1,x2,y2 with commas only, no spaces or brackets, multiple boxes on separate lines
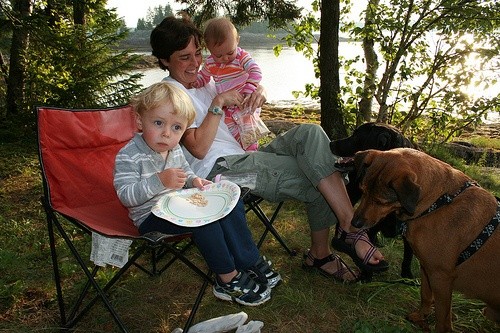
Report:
151,180,241,227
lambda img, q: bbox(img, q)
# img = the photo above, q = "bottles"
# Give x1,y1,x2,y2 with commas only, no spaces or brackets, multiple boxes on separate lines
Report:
212,172,257,190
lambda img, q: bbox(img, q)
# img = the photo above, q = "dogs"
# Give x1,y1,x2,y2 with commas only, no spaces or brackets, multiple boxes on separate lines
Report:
351,146,499,333
329,123,418,279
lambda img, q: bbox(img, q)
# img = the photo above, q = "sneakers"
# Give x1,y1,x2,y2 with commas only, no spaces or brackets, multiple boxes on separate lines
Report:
212,272,272,306
245,256,282,288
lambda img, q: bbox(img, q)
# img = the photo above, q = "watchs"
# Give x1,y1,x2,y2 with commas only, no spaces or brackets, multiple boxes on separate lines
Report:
208,106,223,115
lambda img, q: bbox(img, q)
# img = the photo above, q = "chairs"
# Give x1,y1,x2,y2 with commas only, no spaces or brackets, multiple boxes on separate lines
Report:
35,106,296,333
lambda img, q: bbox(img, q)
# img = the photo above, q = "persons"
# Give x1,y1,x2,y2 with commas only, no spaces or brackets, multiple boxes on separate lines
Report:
149,17,386,283
113,82,282,306
193,18,263,152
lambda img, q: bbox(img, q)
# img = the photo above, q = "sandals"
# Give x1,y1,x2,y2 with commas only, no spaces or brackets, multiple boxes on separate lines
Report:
331,223,390,273
302,250,361,285
